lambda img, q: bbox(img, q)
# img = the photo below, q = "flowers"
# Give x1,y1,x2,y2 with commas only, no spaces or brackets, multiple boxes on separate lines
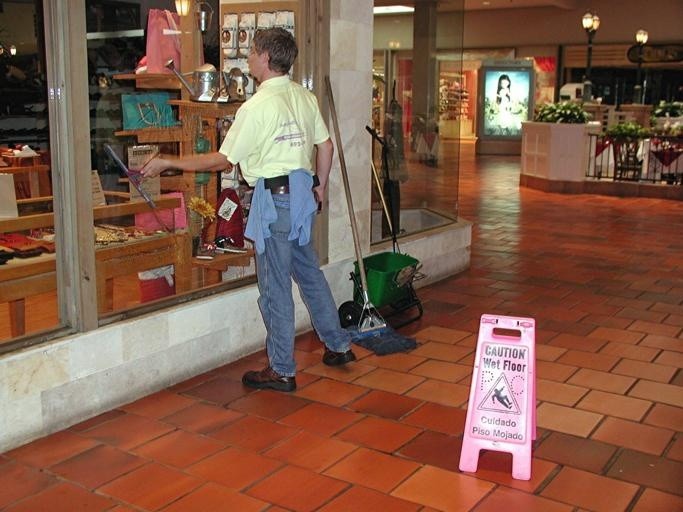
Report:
188,196,217,222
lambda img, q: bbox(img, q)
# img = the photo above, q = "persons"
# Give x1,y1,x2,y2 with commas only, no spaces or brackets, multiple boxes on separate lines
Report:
140,28,356,391
493,75,523,129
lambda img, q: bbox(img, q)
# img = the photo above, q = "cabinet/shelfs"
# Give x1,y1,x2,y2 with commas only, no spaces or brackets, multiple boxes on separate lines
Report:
0,190,193,338
110,1,202,236
165,93,251,274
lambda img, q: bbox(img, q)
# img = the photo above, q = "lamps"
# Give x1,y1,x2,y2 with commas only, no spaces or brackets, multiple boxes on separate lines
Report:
0,44,16,59
174,1,192,17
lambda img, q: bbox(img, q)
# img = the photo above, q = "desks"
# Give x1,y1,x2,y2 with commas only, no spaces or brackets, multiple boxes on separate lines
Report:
592,134,683,184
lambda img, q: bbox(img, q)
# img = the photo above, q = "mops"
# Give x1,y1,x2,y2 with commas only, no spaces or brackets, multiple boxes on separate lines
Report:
321,73,417,356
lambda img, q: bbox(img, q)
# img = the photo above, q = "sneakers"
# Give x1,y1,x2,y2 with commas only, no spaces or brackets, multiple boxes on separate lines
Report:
323,351,355,365
242,366,297,392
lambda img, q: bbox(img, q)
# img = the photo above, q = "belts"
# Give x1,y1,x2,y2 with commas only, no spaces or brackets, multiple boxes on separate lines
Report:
271,186,289,194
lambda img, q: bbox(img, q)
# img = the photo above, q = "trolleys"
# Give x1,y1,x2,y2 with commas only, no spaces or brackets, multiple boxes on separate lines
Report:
335,247,429,334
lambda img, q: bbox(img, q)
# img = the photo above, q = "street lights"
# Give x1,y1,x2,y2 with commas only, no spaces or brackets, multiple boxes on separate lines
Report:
580,9,600,105
632,24,651,105
